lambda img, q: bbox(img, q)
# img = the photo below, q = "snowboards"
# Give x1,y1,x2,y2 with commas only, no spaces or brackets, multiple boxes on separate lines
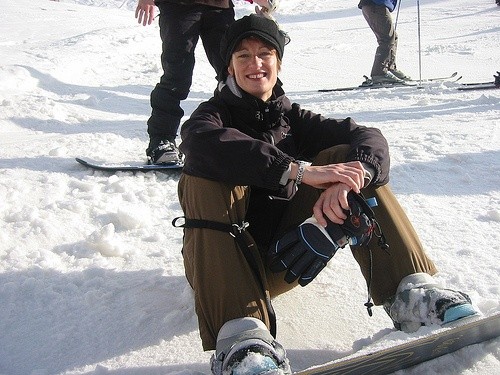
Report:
290,313,500,375
76,155,185,171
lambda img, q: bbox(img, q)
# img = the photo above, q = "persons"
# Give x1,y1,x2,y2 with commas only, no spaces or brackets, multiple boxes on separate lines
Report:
135,0,274,164
177,13,476,375
358,0,406,82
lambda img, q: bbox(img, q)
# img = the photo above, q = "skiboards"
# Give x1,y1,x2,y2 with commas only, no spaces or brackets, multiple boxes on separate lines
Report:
456,80,500,91
318,71,462,92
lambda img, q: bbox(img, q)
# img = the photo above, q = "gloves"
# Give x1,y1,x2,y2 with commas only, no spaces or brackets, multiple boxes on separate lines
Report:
267,188,376,286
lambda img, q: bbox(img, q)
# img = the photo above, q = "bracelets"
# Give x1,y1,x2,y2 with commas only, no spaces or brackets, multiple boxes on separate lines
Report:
295,164,304,185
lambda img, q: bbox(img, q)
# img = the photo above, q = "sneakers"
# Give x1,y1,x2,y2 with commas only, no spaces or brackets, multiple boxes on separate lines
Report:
391,69,409,81
225,347,287,375
147,140,179,166
373,72,404,84
385,288,477,327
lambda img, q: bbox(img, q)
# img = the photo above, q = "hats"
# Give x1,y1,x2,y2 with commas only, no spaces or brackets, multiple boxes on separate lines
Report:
218,16,285,64
